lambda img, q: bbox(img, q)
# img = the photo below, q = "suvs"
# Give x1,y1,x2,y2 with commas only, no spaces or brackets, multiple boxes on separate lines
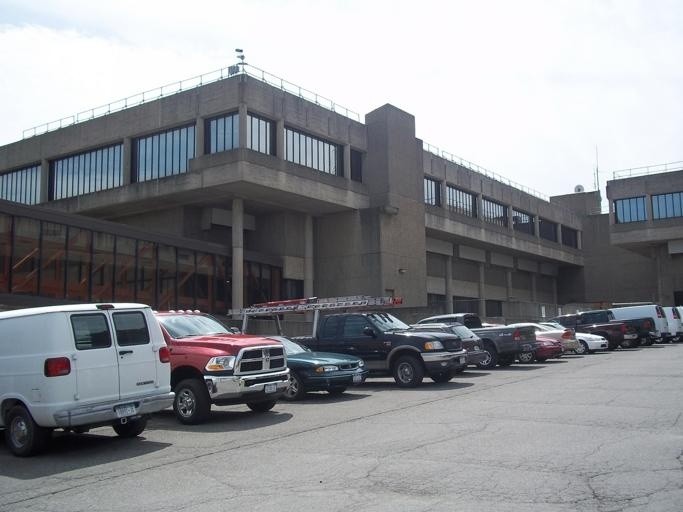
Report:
154,310,291,425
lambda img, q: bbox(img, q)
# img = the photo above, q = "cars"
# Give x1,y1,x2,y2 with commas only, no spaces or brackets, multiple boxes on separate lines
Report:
517,321,609,364
277,335,368,400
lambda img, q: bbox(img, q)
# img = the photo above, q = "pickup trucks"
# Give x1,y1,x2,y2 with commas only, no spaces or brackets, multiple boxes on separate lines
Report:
551,310,657,351
291,312,536,388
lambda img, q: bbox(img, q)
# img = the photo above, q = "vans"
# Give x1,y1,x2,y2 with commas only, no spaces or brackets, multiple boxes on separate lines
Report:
610,305,683,344
0,302,175,457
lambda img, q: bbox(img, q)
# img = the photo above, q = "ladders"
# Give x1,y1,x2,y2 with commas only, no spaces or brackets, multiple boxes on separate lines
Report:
227,295,402,316
